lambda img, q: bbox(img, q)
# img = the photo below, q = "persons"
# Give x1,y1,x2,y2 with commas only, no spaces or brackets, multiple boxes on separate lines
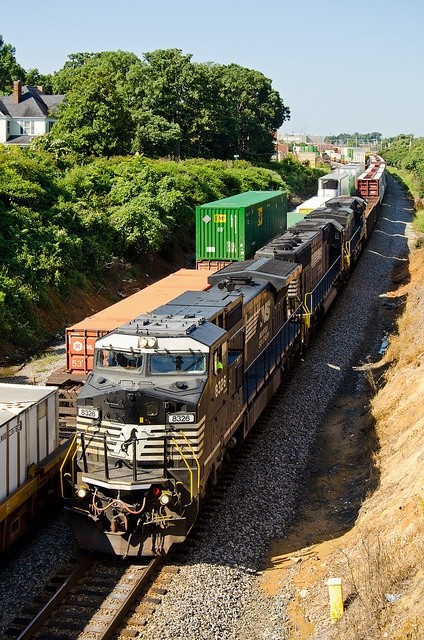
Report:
197,354,223,374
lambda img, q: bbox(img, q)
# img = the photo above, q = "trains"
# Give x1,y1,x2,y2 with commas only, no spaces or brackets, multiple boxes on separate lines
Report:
0,146,368,570
69,154,386,561
